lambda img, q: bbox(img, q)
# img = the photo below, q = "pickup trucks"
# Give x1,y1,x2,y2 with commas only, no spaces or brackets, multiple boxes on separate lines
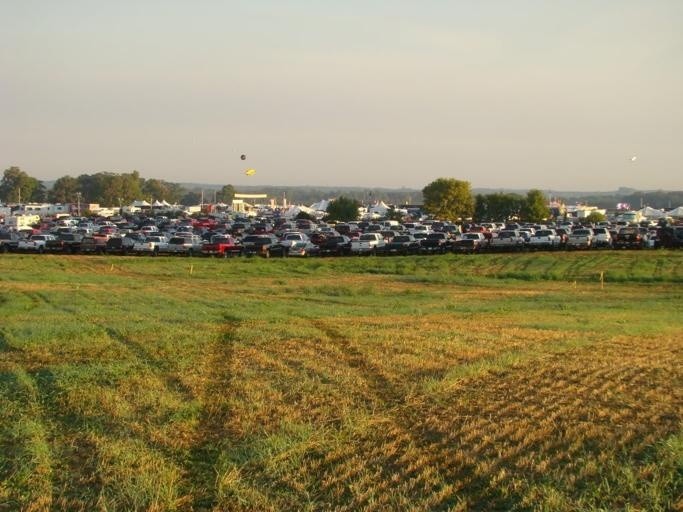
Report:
0,209,683,257
